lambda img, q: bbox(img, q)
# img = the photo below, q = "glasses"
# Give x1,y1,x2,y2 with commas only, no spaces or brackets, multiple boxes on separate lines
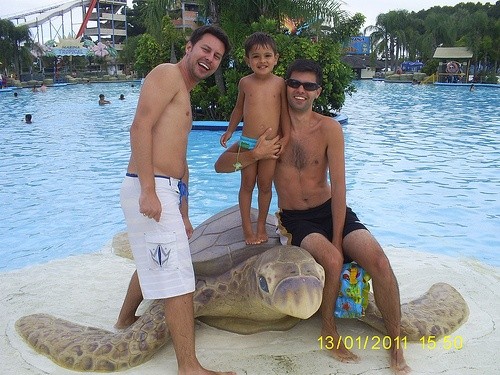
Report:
286,78,322,91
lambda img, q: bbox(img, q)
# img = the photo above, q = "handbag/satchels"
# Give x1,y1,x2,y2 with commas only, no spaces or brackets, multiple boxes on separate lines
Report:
333,261,371,319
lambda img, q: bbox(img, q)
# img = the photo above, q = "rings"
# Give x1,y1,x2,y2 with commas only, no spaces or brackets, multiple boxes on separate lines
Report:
143,212,147,217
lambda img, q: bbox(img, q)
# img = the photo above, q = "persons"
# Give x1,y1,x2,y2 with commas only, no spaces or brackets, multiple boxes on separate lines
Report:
410,78,422,86
219,32,290,245
214,59,412,375
468,84,477,92
0,70,135,126
113,24,237,375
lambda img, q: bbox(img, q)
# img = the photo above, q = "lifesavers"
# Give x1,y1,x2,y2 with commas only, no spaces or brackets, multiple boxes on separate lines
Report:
447,62,458,73
